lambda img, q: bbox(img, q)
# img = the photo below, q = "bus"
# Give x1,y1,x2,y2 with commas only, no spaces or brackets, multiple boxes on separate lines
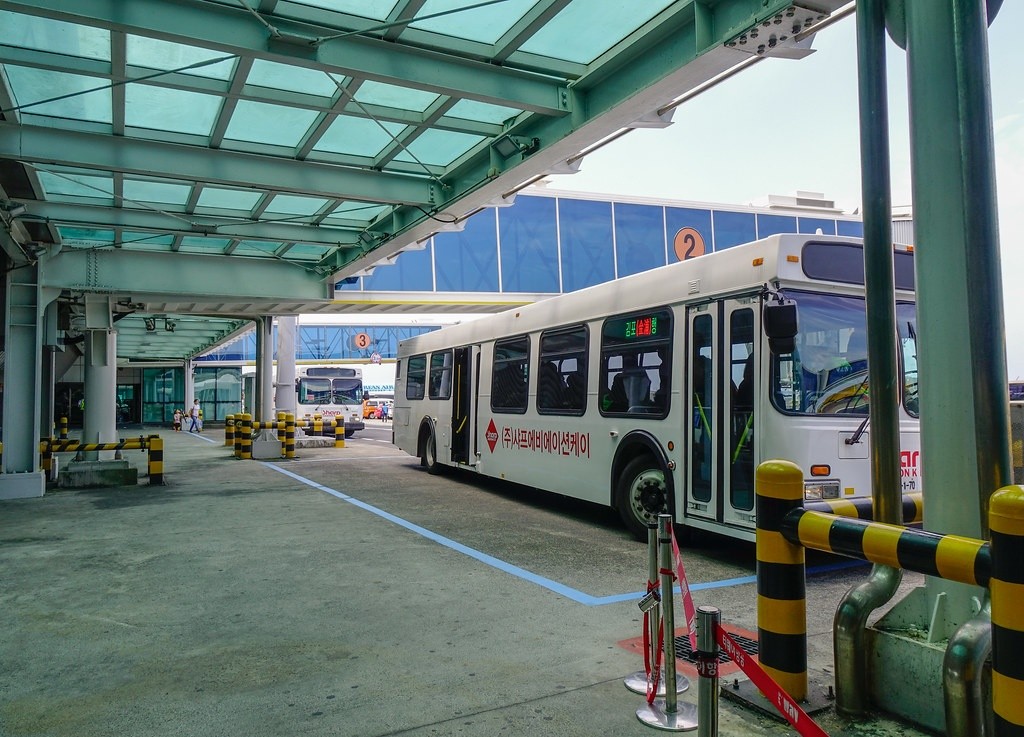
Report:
295,365,370,438
392,233,922,543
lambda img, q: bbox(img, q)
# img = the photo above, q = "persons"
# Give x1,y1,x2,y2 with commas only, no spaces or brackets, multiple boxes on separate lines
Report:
381,404,388,422
189,399,199,432
542,346,752,414
174,409,181,430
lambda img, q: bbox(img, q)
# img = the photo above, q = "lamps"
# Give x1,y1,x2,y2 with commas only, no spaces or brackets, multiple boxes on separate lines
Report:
25,241,47,260
6,201,26,224
360,231,389,243
315,265,335,274
491,134,540,161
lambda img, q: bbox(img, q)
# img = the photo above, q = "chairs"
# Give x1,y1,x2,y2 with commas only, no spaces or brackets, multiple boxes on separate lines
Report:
492,372,649,409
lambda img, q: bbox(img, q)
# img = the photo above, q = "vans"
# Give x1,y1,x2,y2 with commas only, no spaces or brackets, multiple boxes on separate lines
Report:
362,398,394,419
